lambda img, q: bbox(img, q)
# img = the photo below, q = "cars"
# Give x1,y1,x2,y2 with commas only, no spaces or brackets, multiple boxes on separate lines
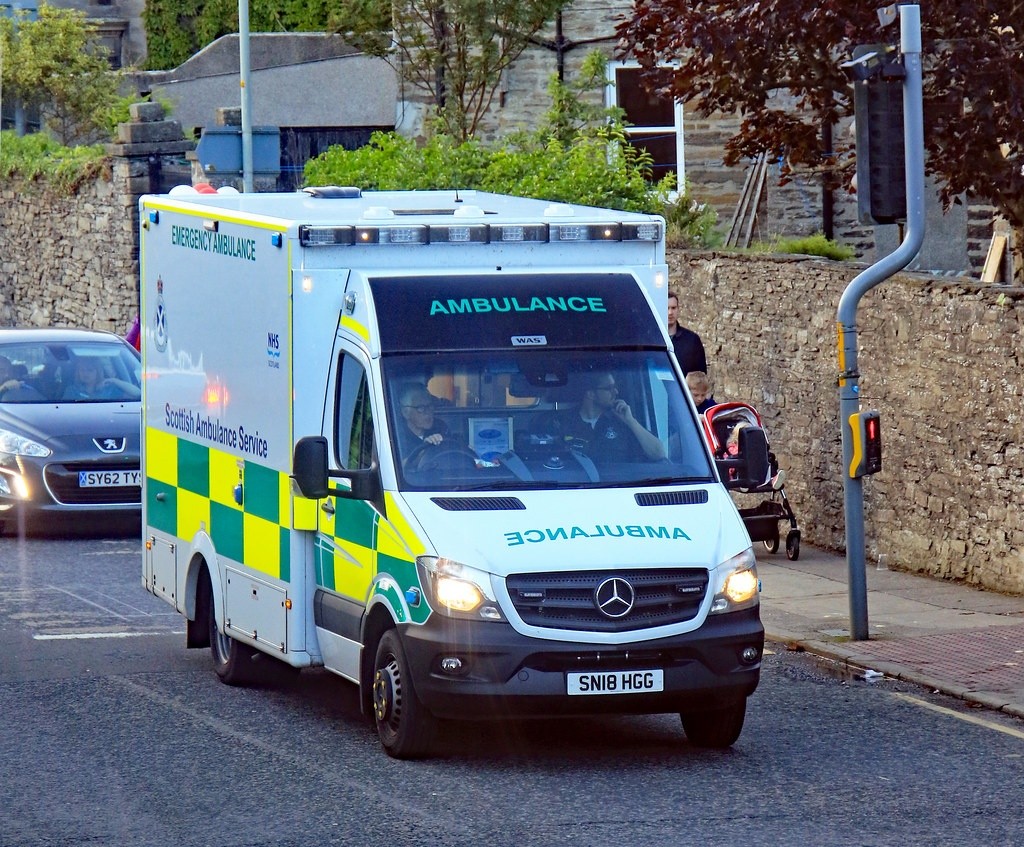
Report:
0,326,141,532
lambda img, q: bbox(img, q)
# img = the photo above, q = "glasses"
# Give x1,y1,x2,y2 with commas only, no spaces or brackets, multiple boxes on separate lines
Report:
595,384,619,392
401,403,434,414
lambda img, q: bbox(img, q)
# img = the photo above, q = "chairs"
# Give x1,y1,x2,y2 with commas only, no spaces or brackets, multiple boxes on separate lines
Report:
532,379,590,436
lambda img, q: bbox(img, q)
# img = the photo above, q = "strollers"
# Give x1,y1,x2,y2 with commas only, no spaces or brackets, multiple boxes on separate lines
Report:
702,402,800,561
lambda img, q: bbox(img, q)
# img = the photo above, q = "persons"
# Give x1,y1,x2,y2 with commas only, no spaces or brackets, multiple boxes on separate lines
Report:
62,356,141,400
538,368,667,462
668,292,708,375
721,420,786,494
371,384,452,471
686,370,718,419
0,355,27,395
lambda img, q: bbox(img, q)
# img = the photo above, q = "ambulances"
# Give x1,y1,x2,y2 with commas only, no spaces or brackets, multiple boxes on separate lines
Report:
140,183,765,760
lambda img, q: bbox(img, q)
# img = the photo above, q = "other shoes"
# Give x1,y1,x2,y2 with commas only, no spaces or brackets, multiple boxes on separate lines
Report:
769,470,786,490
737,472,749,492
